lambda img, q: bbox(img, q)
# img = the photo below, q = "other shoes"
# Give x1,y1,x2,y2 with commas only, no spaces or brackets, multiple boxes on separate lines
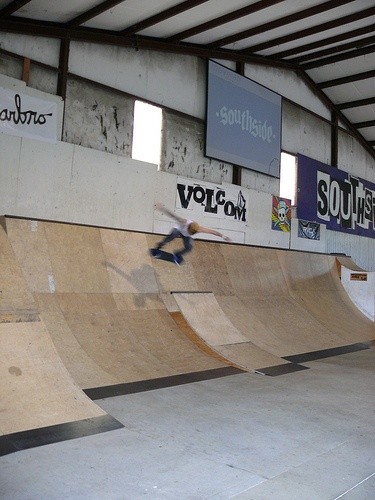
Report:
153,249,157,256
174,252,180,261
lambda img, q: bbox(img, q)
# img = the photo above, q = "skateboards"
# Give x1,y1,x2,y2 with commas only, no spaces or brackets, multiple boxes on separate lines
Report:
150,248,183,264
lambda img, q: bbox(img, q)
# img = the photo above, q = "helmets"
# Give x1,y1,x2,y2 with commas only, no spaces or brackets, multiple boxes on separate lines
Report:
188,223,199,235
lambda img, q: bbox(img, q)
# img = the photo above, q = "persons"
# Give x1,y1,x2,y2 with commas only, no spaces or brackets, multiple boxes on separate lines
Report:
150,202,232,266
